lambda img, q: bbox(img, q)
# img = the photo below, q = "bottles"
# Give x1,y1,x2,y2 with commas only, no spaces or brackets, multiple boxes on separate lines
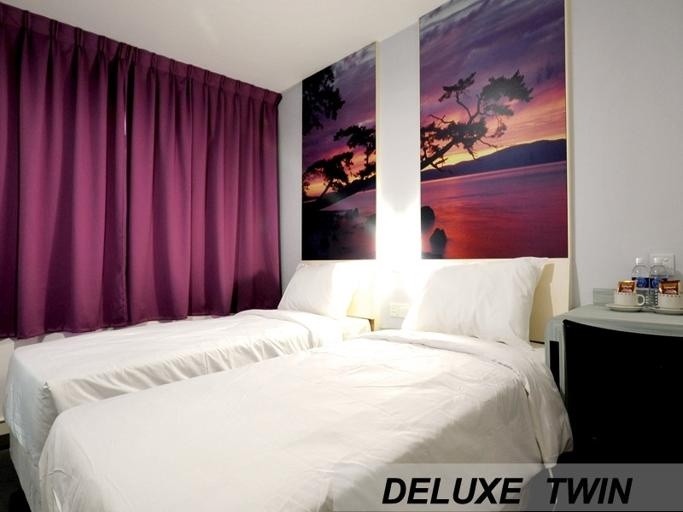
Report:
648,257,667,307
630,258,651,308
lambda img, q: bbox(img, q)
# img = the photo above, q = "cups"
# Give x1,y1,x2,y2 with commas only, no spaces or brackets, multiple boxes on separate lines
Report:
613,291,645,307
656,292,683,309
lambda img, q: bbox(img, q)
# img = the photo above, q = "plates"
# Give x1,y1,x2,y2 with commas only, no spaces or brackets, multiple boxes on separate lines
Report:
605,303,646,312
649,306,683,315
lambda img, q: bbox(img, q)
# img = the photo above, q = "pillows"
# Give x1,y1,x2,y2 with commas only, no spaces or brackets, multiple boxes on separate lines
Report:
400,255,549,349
277,260,364,316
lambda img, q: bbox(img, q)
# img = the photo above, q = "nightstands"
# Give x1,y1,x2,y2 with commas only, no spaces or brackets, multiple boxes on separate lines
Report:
544,302,682,465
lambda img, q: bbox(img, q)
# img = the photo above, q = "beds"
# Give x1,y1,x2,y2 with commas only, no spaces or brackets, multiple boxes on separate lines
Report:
8,310,566,512
